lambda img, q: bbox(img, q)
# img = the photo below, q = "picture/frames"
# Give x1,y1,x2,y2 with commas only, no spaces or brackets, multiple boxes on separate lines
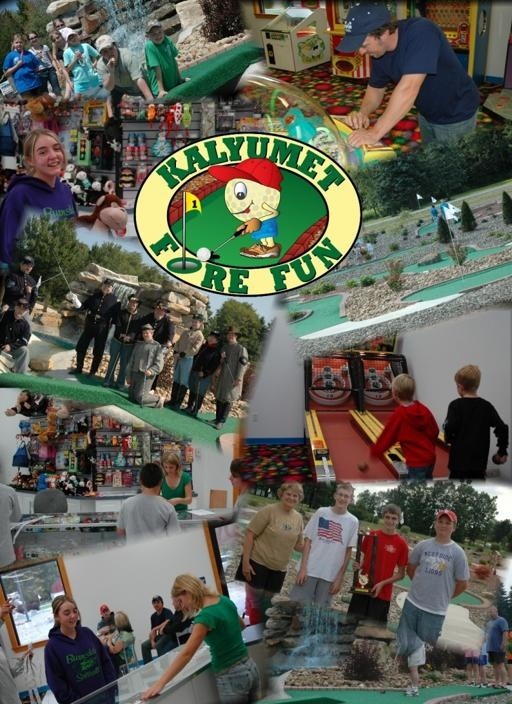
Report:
0,553,80,653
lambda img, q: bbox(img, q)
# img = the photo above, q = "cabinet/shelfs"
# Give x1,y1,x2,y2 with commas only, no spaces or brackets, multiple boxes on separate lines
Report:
13,508,236,562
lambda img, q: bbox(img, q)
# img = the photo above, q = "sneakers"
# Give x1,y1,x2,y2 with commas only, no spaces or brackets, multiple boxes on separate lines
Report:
466,679,512,689
239,243,282,259
405,685,419,697
69,368,225,430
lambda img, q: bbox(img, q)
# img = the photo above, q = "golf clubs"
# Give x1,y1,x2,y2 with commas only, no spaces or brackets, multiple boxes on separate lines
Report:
192,364,204,417
211,225,248,257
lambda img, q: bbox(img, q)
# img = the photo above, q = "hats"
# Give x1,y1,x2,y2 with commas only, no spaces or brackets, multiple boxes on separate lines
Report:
103,277,243,340
436,509,457,525
100,604,109,614
206,158,284,193
152,595,163,604
15,255,34,309
61,18,164,54
335,3,390,52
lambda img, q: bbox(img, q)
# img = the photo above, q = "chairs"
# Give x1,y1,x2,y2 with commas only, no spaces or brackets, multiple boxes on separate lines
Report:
119,636,140,677
33,487,67,514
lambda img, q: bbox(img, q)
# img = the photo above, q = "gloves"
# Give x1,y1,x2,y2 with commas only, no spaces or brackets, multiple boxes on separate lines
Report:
237,218,262,237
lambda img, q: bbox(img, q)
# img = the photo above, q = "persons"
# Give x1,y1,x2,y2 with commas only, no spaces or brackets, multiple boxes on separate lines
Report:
0,0,512,704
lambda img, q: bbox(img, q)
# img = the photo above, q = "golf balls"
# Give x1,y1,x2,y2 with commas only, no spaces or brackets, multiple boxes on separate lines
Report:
196,247,212,261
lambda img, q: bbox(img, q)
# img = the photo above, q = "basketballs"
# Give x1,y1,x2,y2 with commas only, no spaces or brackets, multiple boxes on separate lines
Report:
491,453,500,464
357,460,368,471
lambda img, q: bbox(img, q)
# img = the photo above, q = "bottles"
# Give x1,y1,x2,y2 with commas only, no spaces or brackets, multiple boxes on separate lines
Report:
96,468,133,488
98,453,111,465
125,131,147,162
125,451,142,466
